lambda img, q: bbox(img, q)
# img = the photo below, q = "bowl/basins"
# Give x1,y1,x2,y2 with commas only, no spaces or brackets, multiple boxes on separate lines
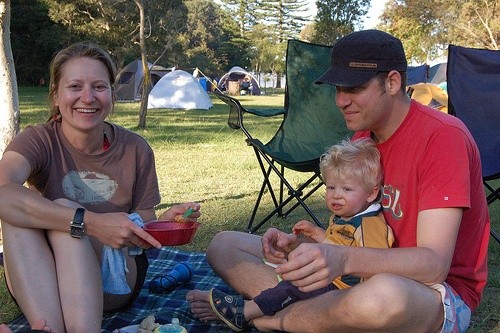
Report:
142,220,201,246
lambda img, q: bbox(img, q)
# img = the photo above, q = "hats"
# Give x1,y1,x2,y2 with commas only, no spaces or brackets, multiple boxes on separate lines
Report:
314,29,408,89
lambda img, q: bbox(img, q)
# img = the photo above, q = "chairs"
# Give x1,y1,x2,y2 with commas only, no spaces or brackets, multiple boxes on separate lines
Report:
212,38,500,245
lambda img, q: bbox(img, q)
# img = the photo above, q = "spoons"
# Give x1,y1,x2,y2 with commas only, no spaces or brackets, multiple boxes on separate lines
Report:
174,208,194,221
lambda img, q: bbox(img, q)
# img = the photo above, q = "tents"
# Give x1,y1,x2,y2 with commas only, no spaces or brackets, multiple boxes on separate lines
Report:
111,59,260,110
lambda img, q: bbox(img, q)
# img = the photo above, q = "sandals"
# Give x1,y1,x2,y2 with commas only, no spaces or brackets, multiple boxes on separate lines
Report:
210,287,251,331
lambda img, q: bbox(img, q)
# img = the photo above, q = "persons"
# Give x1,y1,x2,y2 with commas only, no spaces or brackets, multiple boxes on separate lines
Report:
184,28,489,333
0,40,201,333
211,139,400,333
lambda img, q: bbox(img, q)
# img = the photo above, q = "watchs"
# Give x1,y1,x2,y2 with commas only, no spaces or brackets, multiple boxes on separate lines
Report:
67,208,86,240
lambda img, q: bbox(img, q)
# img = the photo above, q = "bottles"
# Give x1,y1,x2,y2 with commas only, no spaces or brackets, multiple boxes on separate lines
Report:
407,86,414,98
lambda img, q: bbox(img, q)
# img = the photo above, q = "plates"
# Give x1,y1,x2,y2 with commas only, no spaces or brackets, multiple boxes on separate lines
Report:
113,324,164,333
